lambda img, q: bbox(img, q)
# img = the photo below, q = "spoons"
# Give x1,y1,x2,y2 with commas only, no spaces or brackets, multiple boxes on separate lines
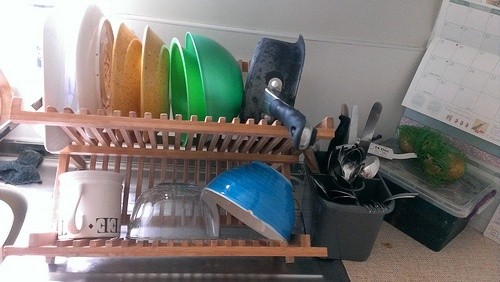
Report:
306,145,381,201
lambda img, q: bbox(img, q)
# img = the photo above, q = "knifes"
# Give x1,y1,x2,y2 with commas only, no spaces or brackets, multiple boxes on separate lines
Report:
325,98,385,157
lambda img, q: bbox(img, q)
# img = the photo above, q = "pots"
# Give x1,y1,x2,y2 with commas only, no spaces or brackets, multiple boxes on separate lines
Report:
240,34,317,151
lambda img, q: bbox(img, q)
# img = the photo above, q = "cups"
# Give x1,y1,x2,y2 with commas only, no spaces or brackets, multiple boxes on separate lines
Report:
56,169,121,241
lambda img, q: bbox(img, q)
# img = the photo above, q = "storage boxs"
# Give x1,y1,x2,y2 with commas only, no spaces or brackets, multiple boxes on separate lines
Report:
362,138,497,251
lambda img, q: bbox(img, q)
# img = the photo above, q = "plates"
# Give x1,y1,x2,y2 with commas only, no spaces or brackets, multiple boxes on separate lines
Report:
141,25,174,142
43,18,73,154
114,19,138,146
76,5,113,140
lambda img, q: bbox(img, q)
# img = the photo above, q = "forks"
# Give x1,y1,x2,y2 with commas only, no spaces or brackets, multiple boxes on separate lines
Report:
362,201,391,215
381,190,420,204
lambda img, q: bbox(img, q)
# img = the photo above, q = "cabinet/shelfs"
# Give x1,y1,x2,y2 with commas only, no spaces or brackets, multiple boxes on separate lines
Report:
1,58,331,265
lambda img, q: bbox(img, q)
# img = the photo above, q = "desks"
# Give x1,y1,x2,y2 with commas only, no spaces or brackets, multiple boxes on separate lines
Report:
0,139,500,282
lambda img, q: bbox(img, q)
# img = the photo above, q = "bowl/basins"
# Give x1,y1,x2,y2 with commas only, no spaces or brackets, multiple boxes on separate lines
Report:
124,178,221,239
167,37,205,147
204,163,298,246
184,31,244,146
